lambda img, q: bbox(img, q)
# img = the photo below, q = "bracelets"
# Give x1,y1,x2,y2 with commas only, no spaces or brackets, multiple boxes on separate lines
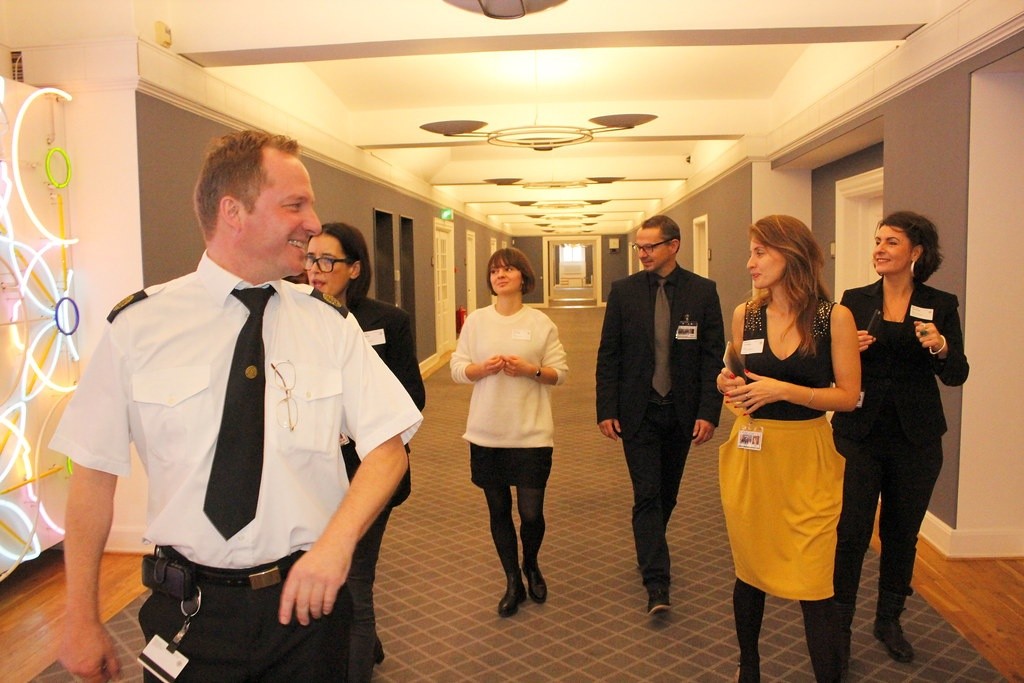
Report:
806,389,814,406
718,386,724,395
929,335,946,354
536,371,541,376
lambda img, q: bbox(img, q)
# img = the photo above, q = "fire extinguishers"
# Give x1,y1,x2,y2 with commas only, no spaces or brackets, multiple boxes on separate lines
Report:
456,305,466,334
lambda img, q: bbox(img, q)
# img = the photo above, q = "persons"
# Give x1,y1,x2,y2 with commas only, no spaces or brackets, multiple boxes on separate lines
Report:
306,223,426,682
449,249,569,619
47,131,424,683
596,215,725,614
717,215,862,683
831,211,969,683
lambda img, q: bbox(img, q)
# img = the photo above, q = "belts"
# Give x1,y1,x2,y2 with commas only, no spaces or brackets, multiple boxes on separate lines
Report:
155,548,300,589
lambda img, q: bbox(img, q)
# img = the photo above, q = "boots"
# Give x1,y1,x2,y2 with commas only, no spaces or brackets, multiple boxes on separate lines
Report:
497,568,527,617
521,552,547,605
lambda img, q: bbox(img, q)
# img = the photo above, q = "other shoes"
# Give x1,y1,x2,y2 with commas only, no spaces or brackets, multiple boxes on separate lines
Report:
647,588,671,615
872,615,914,663
836,629,854,676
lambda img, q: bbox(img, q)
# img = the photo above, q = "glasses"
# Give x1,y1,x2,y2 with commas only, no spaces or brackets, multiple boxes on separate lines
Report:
304,256,349,273
268,357,301,433
632,240,670,253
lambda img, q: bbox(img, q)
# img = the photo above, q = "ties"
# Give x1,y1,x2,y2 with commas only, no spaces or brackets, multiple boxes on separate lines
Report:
651,279,673,397
197,285,278,541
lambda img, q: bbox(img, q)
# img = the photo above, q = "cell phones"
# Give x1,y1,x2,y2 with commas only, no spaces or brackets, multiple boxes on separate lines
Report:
724,341,747,384
867,309,884,336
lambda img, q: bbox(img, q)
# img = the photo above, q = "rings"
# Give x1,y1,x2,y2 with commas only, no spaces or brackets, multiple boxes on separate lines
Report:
746,394,748,398
921,331,926,335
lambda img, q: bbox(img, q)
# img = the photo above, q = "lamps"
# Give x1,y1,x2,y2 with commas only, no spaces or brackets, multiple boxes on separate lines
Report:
481,176,630,234
443,0,566,20
420,114,664,151
